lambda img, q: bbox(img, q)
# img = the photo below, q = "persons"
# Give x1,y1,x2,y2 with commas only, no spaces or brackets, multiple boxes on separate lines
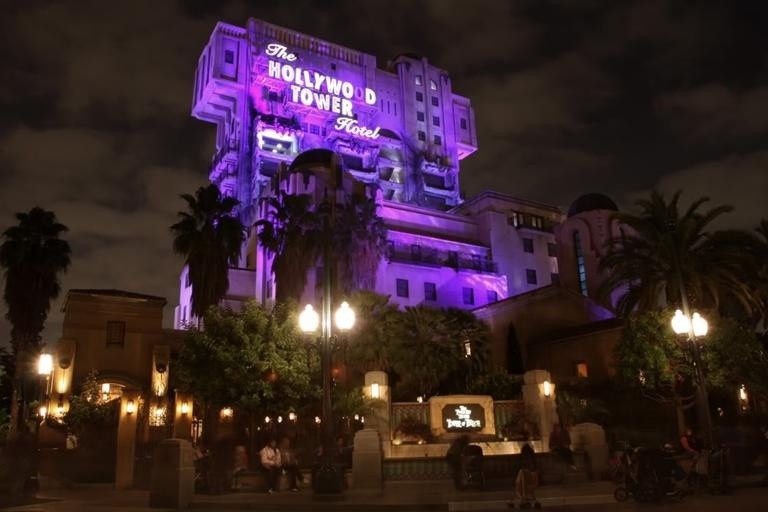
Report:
558,423,577,470
680,425,697,479
192,434,345,495
548,423,561,452
659,431,680,470
447,435,473,490
510,443,543,493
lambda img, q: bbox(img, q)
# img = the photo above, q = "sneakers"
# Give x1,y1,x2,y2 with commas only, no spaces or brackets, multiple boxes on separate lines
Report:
291,478,310,491
267,487,280,493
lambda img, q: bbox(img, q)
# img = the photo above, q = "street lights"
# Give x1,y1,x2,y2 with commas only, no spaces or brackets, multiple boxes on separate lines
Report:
21,352,54,497
297,300,356,502
671,308,717,450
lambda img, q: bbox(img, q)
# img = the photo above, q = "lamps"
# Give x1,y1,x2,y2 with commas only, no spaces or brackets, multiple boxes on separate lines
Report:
153,345,169,372
58,339,76,368
